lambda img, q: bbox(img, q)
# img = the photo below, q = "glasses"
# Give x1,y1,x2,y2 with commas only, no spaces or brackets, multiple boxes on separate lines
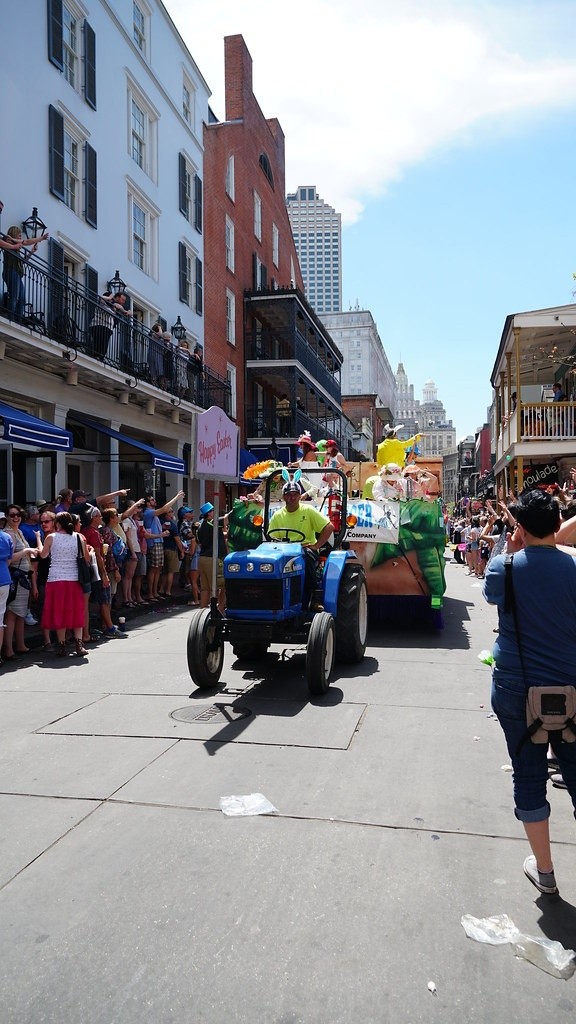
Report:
39,519,55,525
186,510,192,513
0,518,6,521
99,500,174,519
6,513,23,518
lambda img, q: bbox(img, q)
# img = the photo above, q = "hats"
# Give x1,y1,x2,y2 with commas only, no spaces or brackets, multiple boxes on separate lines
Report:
199,502,214,515
378,463,404,481
402,465,421,476
37,500,47,510
296,436,339,451
0,512,5,518
180,506,194,516
72,490,92,501
405,445,420,454
281,468,301,496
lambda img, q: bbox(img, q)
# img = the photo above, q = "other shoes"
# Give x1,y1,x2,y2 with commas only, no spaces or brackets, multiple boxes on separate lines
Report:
311,601,324,612
184,582,210,609
524,854,557,893
547,744,569,789
0,613,129,668
455,561,485,579
124,589,173,608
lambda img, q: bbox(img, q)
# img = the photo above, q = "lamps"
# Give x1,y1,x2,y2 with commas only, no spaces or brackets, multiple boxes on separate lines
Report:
170,316,186,341
145,400,156,415
67,369,78,386
21,207,47,240
107,270,127,295
172,410,180,423
118,390,130,405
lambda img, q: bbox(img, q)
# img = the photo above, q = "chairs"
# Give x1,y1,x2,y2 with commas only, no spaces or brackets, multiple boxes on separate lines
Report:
23,303,46,334
121,350,147,380
57,316,90,352
250,417,267,438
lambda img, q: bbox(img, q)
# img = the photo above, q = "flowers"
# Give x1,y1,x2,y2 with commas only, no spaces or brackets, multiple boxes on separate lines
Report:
380,465,402,475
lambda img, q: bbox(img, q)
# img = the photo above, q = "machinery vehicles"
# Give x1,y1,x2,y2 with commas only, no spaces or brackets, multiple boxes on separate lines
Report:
187,468,369,697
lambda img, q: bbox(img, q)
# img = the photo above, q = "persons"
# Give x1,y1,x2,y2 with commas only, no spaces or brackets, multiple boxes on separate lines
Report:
0,201,23,250
90,291,134,361
3,226,49,326
404,437,420,467
276,394,306,437
313,440,350,497
501,383,576,442
267,482,335,612
0,463,576,661
148,323,203,399
484,489,576,893
289,435,322,492
376,423,430,468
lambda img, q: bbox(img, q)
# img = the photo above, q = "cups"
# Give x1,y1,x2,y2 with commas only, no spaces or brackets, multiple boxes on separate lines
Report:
103,544,109,555
118,616,125,632
30,551,38,558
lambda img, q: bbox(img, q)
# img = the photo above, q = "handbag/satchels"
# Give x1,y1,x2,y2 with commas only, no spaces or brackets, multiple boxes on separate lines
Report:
524,687,576,744
75,534,92,584
